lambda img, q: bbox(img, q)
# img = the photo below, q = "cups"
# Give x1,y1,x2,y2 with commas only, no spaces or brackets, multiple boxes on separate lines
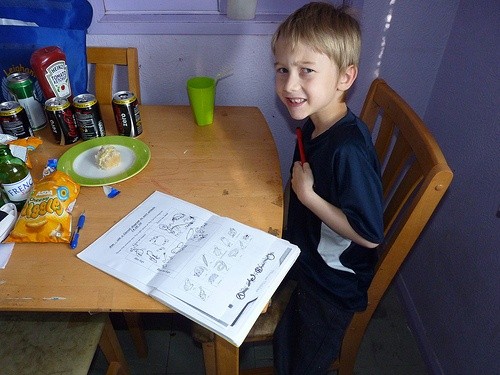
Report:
186,77,216,126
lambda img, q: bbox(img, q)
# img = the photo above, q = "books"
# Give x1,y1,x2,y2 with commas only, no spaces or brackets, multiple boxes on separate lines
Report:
78,191,302,348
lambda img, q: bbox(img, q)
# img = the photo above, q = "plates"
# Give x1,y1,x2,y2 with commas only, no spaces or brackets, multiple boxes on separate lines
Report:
57,135,151,187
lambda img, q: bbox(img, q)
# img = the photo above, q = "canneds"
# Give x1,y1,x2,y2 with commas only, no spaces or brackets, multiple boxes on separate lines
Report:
45,97,79,145
112,91,144,137
7,72,49,131
72,94,107,141
0,101,35,139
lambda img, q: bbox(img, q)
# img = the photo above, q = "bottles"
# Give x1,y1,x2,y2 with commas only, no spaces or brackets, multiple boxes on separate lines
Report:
30,46,77,127
0,145,34,213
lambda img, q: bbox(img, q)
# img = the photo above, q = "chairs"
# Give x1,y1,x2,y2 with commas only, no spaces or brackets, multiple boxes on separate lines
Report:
87,46,150,359
0,312,131,375
194,79,454,375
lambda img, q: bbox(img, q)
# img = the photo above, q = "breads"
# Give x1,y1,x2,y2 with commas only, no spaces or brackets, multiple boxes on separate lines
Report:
95,145,121,170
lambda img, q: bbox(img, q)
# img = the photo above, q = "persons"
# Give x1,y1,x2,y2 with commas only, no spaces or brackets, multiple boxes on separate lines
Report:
271,1,385,375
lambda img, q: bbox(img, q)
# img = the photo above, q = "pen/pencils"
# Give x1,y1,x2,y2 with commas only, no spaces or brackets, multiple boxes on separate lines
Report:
69,208,86,249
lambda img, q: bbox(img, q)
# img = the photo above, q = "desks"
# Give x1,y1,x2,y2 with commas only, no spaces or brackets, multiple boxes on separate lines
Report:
0,105,284,375
